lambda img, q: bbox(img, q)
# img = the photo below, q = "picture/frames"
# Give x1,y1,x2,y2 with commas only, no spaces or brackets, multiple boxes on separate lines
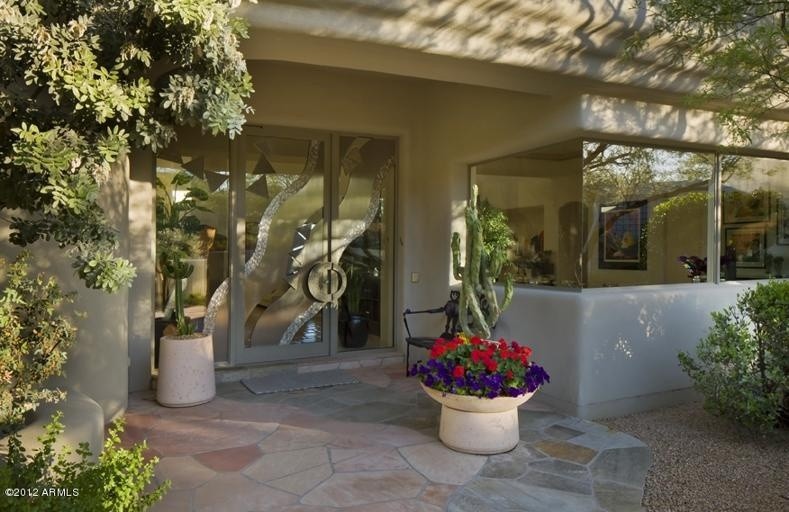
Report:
777,197,789,244
725,226,768,269
598,199,649,271
722,168,772,224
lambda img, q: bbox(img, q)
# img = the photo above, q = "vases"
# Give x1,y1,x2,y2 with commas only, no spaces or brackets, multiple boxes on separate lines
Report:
417,380,541,455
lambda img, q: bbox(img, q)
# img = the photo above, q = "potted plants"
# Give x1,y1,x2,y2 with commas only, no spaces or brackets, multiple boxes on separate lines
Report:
156,250,216,408
339,231,375,349
152,170,217,367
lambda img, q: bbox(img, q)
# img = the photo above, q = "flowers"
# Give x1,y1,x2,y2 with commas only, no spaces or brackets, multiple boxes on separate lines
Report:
406,331,551,400
